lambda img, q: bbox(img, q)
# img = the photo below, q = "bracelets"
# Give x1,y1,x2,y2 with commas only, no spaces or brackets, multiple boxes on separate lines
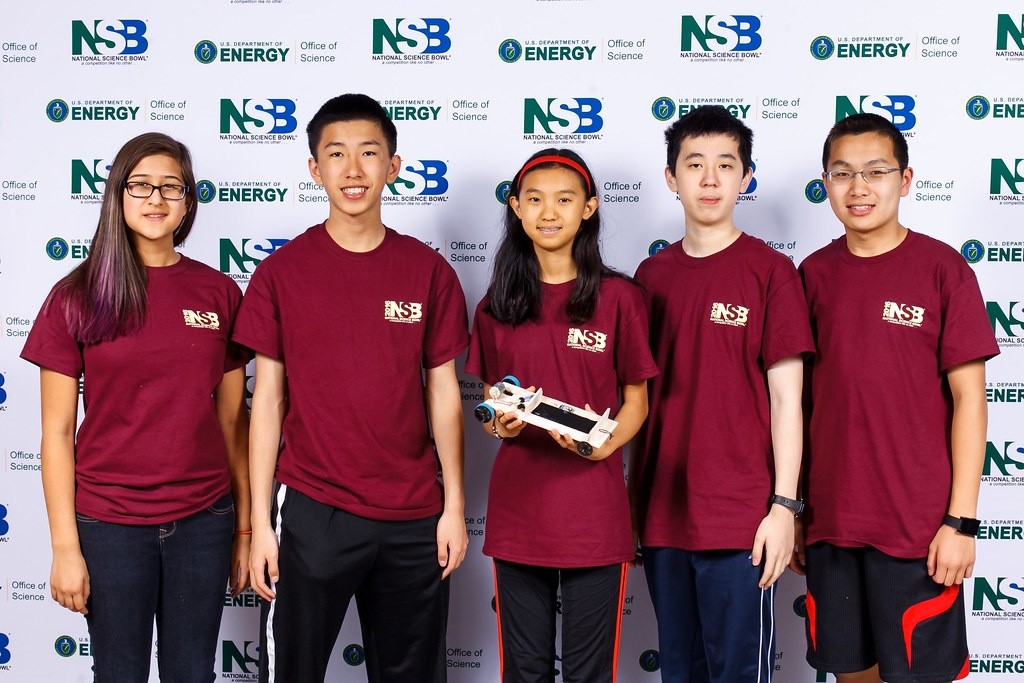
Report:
234,531,252,534
492,418,504,440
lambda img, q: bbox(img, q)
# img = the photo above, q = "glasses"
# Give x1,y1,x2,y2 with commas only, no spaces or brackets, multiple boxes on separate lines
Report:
825,166,901,186
124,181,188,200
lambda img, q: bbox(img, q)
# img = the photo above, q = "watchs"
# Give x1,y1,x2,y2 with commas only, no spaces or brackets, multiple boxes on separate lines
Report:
945,514,981,535
770,495,805,518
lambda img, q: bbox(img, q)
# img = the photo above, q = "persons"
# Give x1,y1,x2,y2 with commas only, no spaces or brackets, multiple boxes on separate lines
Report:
231,94,470,683
20,132,251,683
462,147,661,683
788,113,1000,683
631,106,817,683
